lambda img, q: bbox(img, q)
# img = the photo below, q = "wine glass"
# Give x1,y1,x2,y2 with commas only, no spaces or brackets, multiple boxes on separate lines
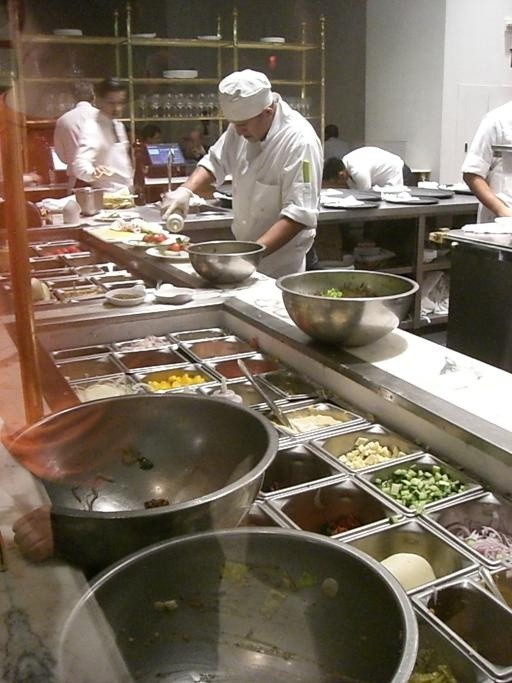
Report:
140,91,315,116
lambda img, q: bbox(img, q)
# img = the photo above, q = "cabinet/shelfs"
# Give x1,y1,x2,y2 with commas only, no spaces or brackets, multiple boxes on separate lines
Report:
306,209,477,330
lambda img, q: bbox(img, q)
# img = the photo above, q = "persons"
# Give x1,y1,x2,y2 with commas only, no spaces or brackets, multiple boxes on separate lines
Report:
323,145,418,264
53,77,219,208
0,418,54,571
324,125,350,161
159,70,323,281
459,98,511,222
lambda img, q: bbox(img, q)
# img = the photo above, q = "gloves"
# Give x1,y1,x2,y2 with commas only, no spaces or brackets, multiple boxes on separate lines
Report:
159,186,194,222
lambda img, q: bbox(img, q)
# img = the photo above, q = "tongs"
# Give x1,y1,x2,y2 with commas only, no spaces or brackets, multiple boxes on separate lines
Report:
235,355,305,427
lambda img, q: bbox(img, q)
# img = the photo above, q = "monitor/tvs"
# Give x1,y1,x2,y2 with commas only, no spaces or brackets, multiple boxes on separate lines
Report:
144,142,188,166
49,145,68,171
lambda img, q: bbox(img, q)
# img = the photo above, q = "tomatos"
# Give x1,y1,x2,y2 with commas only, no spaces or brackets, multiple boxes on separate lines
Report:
169,243,180,251
42,247,81,256
142,233,167,242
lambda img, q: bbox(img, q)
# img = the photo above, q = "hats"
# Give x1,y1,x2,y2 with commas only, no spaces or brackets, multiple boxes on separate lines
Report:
218,68,273,123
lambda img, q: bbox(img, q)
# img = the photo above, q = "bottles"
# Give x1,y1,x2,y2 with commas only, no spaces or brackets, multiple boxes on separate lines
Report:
64,200,79,223
44,209,54,225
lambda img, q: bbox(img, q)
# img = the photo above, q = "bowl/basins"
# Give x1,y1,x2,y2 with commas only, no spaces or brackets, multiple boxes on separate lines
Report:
199,34,222,40
54,28,83,36
7,395,279,576
162,68,202,79
278,266,418,347
261,36,287,43
57,524,421,683
187,237,266,284
131,31,157,38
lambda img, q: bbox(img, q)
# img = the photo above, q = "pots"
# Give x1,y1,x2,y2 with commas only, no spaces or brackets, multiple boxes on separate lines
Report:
75,186,107,215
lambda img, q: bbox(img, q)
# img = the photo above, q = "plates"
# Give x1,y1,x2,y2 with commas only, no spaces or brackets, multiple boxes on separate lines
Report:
91,209,192,258
107,284,194,304
